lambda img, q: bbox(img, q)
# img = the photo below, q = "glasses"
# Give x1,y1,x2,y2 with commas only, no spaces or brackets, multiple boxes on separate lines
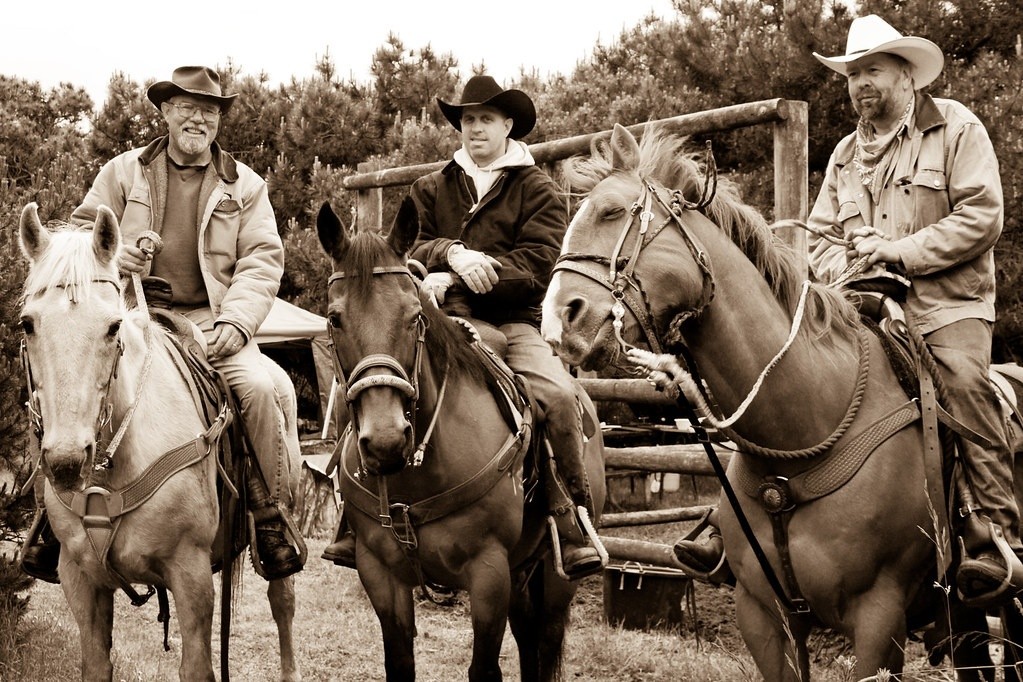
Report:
167,100,223,124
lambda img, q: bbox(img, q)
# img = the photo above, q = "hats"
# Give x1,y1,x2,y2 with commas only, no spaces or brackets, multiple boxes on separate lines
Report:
436,75,536,141
147,66,240,115
813,15,943,91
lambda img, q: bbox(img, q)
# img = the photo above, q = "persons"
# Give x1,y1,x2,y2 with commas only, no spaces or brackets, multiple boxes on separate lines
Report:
23,65,303,584
674,15,1023,610
322,76,603,580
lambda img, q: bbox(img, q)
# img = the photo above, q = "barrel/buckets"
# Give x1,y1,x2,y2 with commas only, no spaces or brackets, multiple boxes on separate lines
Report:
603,560,690,632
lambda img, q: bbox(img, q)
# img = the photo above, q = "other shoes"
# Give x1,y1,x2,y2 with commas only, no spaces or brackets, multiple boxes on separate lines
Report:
322,534,358,562
674,530,737,589
554,543,600,575
958,553,1007,597
22,543,60,582
256,519,297,569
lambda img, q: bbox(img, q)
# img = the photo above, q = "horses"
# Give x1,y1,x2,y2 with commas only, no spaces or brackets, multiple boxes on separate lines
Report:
541,122,1023,682
16,200,300,682
315,194,605,682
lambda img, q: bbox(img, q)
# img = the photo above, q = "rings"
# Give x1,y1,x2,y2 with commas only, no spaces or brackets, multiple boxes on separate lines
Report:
233,344,237,348
